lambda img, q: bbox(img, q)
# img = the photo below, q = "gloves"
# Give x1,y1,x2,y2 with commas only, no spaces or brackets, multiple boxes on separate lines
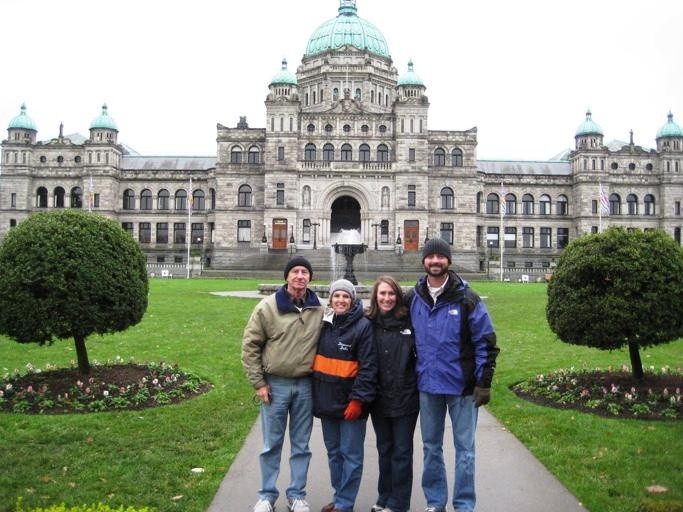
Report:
344,400,363,420
472,386,491,407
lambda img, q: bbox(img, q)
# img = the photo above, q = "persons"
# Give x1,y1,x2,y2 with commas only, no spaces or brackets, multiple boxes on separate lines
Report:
310,275,377,511
241,254,326,512
361,275,422,512
401,240,497,511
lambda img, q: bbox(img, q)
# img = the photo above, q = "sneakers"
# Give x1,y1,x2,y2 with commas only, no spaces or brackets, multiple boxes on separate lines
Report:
253,499,274,512
371,504,383,511
378,507,398,512
426,507,447,512
321,503,334,511
286,498,310,512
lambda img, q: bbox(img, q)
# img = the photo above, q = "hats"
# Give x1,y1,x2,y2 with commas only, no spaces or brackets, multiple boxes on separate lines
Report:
284,256,313,281
423,238,451,263
328,279,357,302
